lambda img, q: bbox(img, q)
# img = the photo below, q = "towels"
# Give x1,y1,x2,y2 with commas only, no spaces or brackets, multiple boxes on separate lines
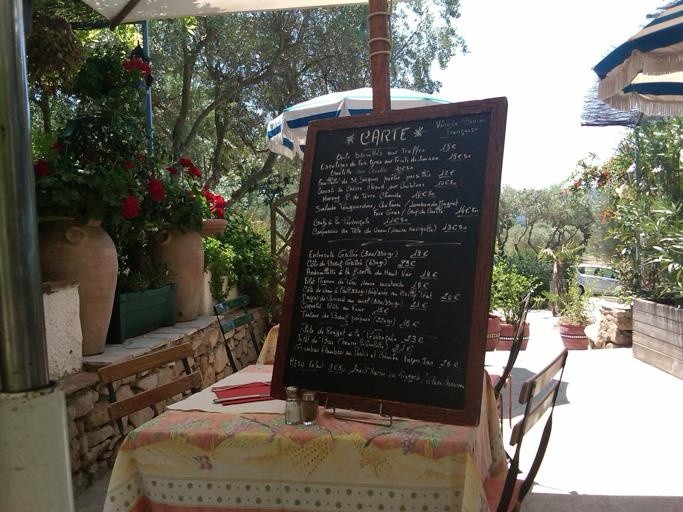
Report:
212,383,286,405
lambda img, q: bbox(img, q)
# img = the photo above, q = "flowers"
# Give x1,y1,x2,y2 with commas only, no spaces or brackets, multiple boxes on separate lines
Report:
35,56,205,228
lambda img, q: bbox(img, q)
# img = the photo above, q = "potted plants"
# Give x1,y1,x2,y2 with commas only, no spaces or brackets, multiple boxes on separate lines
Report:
498,274,530,350
558,262,595,350
485,266,509,350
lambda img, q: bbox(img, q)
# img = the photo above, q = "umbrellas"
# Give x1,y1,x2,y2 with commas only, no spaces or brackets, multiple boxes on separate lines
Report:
591,0,682,116
581,73,661,127
266,85,457,161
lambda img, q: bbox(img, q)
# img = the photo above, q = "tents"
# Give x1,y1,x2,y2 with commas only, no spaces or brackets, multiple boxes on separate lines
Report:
77,0,358,24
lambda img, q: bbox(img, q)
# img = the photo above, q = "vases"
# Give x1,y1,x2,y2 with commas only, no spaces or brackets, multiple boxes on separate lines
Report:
114,286,177,340
49,216,118,353
157,225,207,320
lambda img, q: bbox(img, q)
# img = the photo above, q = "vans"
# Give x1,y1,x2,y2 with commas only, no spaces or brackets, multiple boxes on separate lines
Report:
575,262,620,298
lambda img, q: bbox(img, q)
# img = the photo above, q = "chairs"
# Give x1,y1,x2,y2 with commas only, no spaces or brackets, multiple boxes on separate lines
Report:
100,338,203,420
482,347,569,507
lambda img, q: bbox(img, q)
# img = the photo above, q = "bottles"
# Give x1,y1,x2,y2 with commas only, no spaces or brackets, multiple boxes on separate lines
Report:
284,386,302,424
302,392,319,425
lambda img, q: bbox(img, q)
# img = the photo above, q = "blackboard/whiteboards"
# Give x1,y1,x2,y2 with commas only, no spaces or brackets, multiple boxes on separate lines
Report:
270,96,510,429
